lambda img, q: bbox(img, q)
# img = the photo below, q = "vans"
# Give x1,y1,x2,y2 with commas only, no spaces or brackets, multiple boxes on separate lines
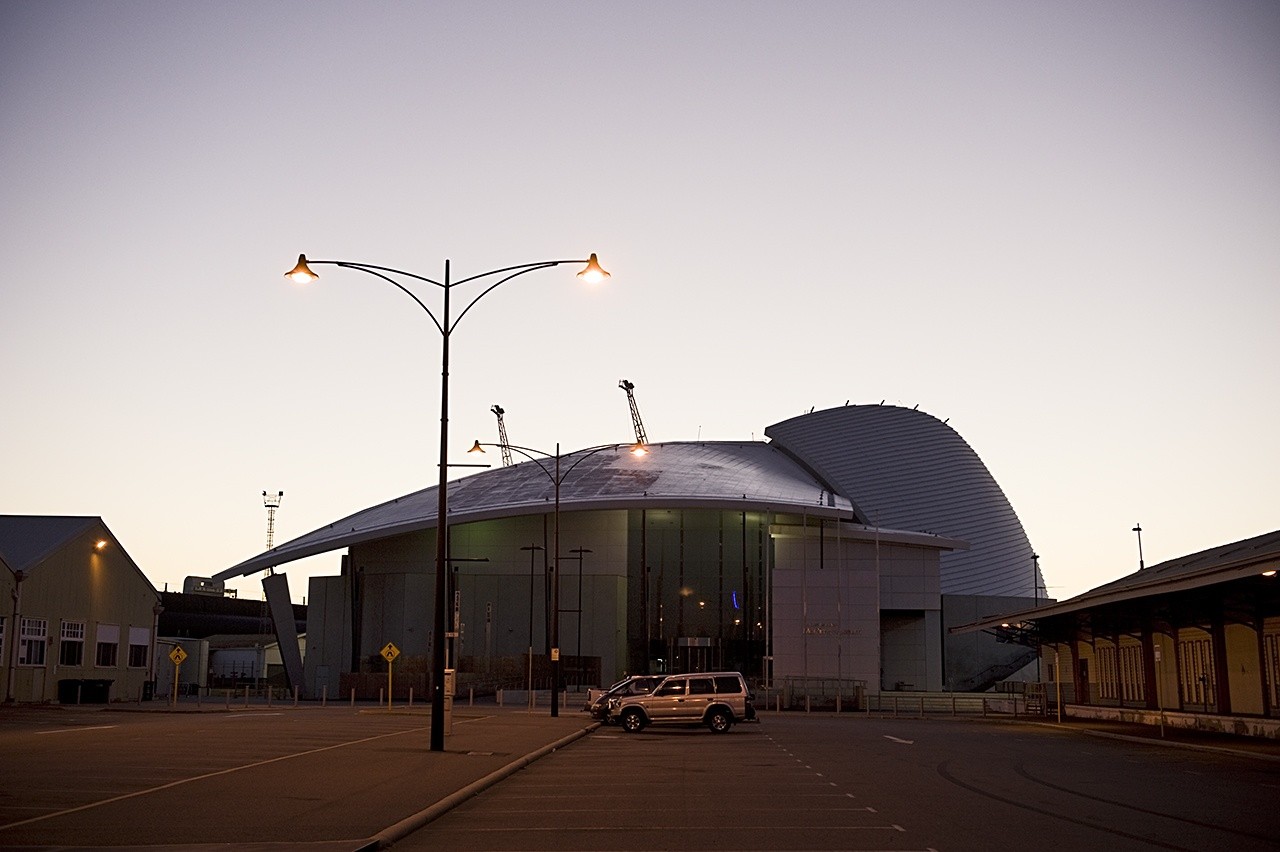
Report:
590,675,670,726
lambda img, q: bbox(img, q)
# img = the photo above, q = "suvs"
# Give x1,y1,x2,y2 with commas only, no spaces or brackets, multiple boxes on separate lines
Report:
608,671,756,733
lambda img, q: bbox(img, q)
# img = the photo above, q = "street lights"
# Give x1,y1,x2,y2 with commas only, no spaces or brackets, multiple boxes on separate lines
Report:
520,543,545,647
467,439,649,717
1030,552,1042,717
568,545,594,694
1131,523,1145,568
283,253,611,751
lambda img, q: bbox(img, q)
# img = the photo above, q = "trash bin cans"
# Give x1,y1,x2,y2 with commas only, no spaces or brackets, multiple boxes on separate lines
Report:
178,682,197,698
142,680,153,701
58,678,113,705
